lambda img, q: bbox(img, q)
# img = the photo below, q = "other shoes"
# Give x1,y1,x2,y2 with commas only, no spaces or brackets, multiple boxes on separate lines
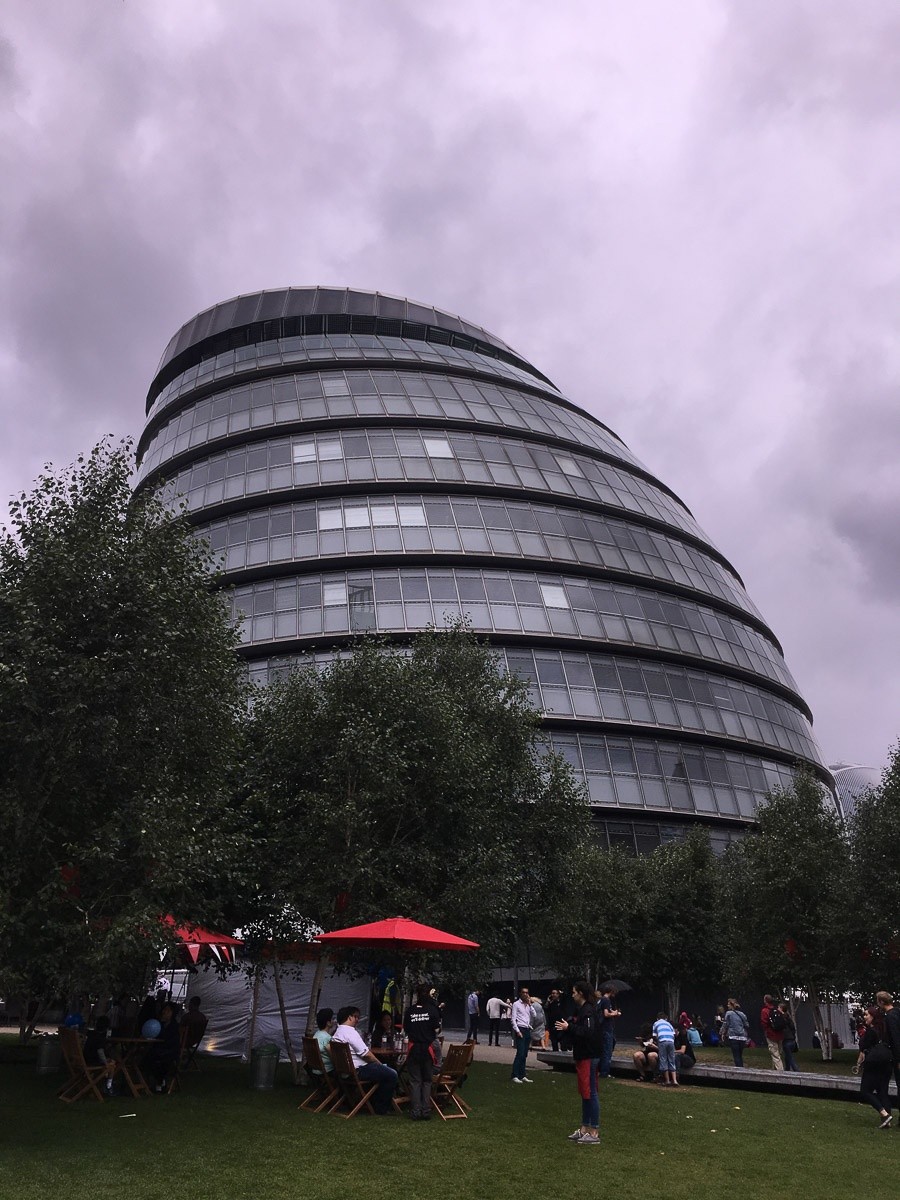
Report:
164,1076,176,1095
672,1080,679,1086
422,1108,433,1119
410,1108,422,1120
661,1081,672,1086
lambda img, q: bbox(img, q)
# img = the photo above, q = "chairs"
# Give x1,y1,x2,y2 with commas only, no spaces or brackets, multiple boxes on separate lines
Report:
430,1038,476,1121
297,1036,341,1114
327,1040,380,1119
55,1019,209,1104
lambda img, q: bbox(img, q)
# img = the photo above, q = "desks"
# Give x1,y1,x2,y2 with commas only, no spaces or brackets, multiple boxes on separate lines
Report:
369,1048,411,1115
106,1038,165,1099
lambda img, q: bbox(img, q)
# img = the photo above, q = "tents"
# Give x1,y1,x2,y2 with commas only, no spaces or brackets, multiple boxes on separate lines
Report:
184,902,372,1064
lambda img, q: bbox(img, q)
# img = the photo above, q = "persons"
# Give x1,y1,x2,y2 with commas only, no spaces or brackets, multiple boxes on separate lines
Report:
462,988,481,1045
503,974,900,1144
311,971,445,1122
857,1008,894,1130
652,1011,680,1087
332,1005,399,1117
511,985,537,1084
403,983,443,1122
485,992,513,1047
82,989,207,1097
554,980,607,1143
719,997,750,1067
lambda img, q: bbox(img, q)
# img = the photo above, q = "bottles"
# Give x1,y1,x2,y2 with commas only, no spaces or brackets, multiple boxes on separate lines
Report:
618,1008,621,1016
361,1032,410,1053
108,1027,112,1038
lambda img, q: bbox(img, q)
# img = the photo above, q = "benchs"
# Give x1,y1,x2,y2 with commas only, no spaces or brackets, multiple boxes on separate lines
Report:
537,1052,897,1096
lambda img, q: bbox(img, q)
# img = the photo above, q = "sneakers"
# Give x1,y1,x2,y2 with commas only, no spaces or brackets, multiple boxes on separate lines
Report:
885,1122,891,1128
568,1129,582,1139
577,1133,601,1144
878,1114,893,1129
512,1077,523,1084
521,1076,533,1083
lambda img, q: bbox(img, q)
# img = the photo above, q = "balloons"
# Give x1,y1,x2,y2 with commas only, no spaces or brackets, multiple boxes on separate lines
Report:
141,1019,161,1038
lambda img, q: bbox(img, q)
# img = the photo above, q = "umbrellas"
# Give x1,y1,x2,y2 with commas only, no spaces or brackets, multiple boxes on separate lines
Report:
597,974,633,1010
144,909,244,1001
312,914,480,1048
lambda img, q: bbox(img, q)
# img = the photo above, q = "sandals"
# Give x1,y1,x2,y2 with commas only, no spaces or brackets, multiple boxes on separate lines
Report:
636,1074,645,1082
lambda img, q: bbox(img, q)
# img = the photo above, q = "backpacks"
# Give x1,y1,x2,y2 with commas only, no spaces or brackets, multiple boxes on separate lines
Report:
868,1042,892,1069
767,1008,788,1032
